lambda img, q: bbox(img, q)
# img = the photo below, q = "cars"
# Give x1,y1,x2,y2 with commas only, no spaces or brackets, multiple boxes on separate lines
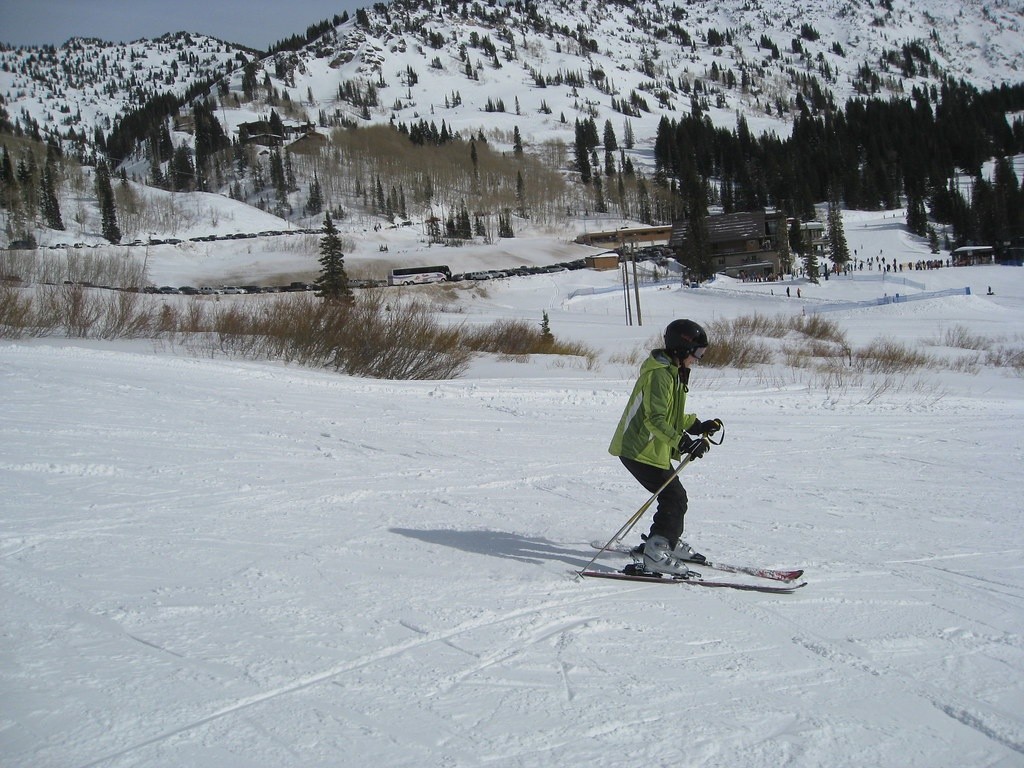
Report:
0,216,442,251
3,275,390,295
452,244,670,283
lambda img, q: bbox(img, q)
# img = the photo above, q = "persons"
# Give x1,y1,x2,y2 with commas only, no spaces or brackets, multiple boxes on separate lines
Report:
740,250,991,297
608,318,722,578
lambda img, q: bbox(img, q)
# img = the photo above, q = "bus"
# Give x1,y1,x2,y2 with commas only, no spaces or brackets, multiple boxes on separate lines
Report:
387,265,452,286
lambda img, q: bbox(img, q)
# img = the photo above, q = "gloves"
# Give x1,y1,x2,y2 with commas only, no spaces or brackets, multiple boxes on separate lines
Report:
678,432,710,461
686,418,720,436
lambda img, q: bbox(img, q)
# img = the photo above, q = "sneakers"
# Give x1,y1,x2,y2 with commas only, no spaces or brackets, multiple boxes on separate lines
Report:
671,539,695,561
641,534,689,575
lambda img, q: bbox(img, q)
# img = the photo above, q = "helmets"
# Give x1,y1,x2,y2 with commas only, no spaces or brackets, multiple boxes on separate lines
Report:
664,319,708,362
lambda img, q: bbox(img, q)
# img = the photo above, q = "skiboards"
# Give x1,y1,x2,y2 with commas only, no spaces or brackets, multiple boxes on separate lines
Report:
577,536,808,594
